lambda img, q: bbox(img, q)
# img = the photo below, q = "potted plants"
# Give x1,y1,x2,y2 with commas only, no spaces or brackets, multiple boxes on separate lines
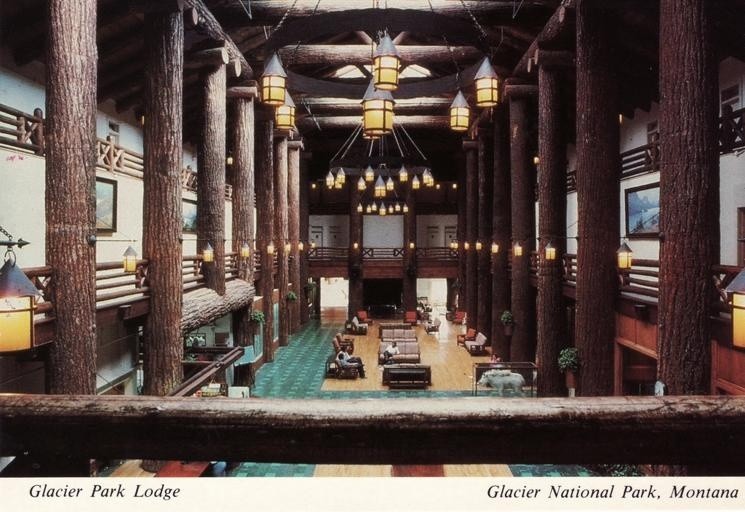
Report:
557,347,577,388
248,307,266,335
500,310,515,336
286,290,297,312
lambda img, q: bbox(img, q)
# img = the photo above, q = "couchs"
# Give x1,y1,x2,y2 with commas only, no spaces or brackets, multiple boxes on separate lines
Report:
424,317,441,335
351,316,368,335
377,322,432,390
335,350,359,379
464,331,487,356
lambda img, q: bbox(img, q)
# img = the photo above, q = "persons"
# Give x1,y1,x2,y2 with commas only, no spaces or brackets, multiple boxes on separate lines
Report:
337,346,368,379
490,353,503,363
384,342,400,361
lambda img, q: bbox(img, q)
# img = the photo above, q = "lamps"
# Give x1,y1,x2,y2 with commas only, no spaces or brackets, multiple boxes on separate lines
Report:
85,215,141,274
0,225,40,357
177,219,316,263
254,0,504,218
449,212,666,270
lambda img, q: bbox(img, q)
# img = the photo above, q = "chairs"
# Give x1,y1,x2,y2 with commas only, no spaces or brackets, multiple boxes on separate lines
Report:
457,328,477,345
451,311,466,327
403,310,428,326
332,332,354,355
357,310,374,326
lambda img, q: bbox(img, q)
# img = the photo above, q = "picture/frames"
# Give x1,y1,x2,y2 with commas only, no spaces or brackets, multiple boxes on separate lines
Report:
95,175,119,233
182,196,197,233
624,180,661,239
